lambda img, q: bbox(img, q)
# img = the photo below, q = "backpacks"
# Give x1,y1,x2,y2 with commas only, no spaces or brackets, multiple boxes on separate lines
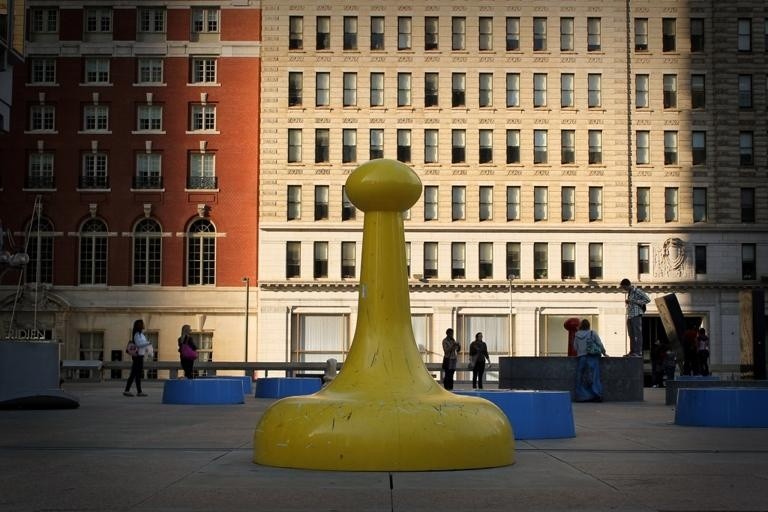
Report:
635,288,646,312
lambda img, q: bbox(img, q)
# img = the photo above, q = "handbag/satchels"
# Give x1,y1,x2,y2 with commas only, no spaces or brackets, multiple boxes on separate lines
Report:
443,357,450,370
588,332,600,353
181,344,198,358
126,341,138,354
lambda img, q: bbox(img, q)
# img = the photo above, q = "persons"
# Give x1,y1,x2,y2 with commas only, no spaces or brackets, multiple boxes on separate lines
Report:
123,319,153,397
573,319,608,402
651,328,712,388
442,328,461,390
178,324,199,379
469,332,491,389
620,278,650,359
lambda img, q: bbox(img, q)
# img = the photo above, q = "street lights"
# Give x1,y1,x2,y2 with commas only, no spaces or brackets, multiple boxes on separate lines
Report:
240,275,252,376
507,273,516,358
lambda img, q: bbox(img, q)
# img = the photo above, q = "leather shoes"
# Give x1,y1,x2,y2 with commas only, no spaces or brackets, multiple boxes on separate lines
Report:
597,396,604,402
137,393,147,397
123,392,134,397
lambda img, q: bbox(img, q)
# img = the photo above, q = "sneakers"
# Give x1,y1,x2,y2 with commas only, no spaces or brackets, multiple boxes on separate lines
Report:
623,352,642,358
